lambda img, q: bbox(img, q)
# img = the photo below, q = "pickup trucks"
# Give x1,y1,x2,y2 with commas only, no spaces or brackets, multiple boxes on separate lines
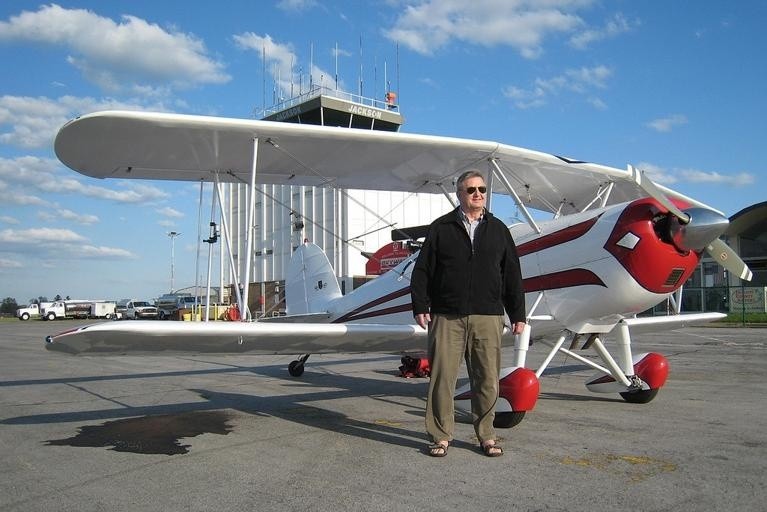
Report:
114,305,128,314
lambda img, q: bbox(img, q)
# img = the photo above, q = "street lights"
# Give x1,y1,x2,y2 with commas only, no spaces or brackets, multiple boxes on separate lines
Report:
166,231,181,296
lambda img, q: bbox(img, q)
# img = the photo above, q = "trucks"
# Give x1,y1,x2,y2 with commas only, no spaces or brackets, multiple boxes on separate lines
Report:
156,293,201,319
41,302,92,321
126,297,158,320
15,302,53,321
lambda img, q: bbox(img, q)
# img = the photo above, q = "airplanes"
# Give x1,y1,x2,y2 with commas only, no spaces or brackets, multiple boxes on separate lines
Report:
42,109,753,428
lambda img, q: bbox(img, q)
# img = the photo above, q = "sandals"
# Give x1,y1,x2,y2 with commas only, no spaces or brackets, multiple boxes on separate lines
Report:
429,440,449,457
480,441,504,456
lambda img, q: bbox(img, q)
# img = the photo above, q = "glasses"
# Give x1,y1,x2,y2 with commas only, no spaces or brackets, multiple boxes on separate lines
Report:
459,186,487,194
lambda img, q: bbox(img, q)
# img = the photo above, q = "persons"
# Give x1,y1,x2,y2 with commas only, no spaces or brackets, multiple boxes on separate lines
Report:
410,171,526,457
